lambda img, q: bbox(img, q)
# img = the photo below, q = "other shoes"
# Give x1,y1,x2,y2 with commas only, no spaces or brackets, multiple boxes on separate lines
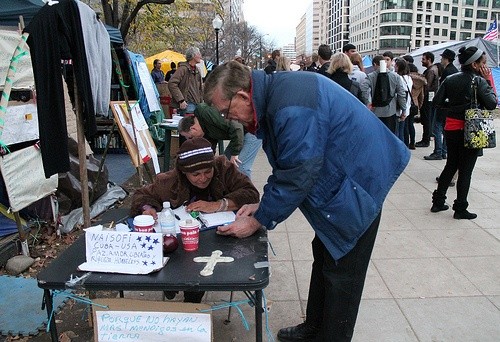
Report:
442,152,448,159
163,291,179,300
423,152,442,160
409,143,415,149
416,140,430,147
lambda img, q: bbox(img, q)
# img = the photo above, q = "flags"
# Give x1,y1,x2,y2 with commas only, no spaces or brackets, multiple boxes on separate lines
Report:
483,14,498,43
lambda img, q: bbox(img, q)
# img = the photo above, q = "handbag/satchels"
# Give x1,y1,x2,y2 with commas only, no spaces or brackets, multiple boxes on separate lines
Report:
463,75,497,148
411,103,420,116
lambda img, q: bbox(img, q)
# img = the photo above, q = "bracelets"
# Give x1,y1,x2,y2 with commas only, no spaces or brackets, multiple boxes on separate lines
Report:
217,200,224,211
222,198,228,211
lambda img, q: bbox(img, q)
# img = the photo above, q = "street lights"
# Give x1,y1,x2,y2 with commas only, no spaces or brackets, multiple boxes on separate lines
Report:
260,34,270,69
212,13,224,65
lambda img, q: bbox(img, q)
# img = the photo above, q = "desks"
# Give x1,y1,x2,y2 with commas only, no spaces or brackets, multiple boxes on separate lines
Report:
37,209,268,342
160,116,224,172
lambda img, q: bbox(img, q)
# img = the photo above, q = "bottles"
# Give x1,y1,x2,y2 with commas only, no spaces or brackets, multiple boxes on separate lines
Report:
159,201,178,252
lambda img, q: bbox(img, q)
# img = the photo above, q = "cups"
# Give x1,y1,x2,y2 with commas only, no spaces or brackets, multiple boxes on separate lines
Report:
172,114,180,124
132,215,155,233
102,223,116,231
178,220,199,251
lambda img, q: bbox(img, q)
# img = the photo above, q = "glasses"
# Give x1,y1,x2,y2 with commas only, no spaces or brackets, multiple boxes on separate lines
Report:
218,96,233,121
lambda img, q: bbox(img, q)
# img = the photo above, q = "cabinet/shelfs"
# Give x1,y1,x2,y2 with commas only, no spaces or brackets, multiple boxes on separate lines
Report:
92,84,123,154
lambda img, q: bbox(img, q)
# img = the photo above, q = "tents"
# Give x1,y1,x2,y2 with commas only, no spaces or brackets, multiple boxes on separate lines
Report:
0,0,155,260
144,49,208,81
404,37,500,79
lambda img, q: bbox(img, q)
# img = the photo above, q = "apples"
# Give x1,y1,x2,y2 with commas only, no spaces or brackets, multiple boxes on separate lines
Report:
163,234,179,251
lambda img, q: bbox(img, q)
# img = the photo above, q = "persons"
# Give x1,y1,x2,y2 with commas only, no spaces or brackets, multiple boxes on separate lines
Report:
366,56,407,134
203,64,217,83
151,59,165,83
235,57,242,63
424,49,459,160
430,46,498,220
264,44,444,150
166,62,177,82
169,46,204,116
128,138,260,302
415,52,439,147
203,60,411,342
178,102,262,178
327,52,363,102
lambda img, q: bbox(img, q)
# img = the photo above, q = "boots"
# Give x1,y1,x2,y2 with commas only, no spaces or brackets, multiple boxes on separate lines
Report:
430,190,448,212
451,199,477,219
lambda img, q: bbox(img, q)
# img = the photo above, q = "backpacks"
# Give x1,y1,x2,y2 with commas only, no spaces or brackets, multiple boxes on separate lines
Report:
372,72,394,107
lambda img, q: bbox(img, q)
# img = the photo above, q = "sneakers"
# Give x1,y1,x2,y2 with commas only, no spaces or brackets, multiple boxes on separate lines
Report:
277,320,320,342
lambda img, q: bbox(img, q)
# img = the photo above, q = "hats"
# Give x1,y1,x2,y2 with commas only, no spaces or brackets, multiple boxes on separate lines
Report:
175,137,215,173
